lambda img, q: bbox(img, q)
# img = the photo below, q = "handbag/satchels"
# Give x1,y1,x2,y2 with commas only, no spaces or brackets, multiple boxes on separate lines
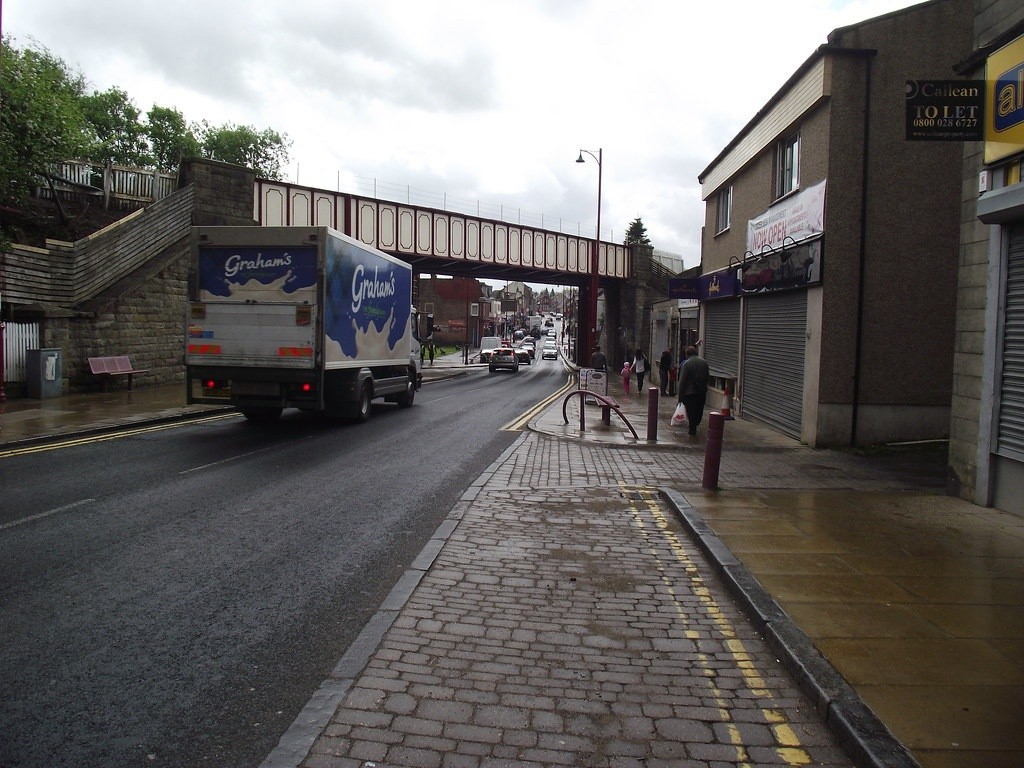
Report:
671,402,689,428
644,356,651,373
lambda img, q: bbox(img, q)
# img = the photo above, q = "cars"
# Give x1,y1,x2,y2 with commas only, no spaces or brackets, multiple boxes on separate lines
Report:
488,348,519,373
479,312,561,364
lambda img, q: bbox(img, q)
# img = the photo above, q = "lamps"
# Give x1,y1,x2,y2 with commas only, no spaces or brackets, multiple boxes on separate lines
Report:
757,244,779,264
741,251,760,273
779,237,800,264
727,256,742,275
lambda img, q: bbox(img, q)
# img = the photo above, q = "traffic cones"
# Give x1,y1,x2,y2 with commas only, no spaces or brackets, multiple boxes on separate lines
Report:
717,388,736,421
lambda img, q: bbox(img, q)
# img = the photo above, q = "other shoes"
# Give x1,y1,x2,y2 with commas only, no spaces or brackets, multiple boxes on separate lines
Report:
669,394,677,397
660,392,669,397
688,430,697,435
637,392,641,394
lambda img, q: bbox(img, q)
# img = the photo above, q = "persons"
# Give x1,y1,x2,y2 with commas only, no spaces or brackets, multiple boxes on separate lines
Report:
620,362,633,393
428,342,437,364
590,345,607,371
678,346,709,436
659,346,677,397
420,342,425,365
629,349,647,394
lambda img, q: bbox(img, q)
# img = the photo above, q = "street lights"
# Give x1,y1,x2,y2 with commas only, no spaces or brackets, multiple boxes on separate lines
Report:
574,148,603,354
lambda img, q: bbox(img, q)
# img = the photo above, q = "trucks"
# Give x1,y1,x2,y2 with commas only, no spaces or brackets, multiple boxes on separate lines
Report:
182,224,434,426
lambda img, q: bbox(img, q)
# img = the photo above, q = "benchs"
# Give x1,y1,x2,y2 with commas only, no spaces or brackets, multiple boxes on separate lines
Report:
595,396,620,425
87,357,149,392
